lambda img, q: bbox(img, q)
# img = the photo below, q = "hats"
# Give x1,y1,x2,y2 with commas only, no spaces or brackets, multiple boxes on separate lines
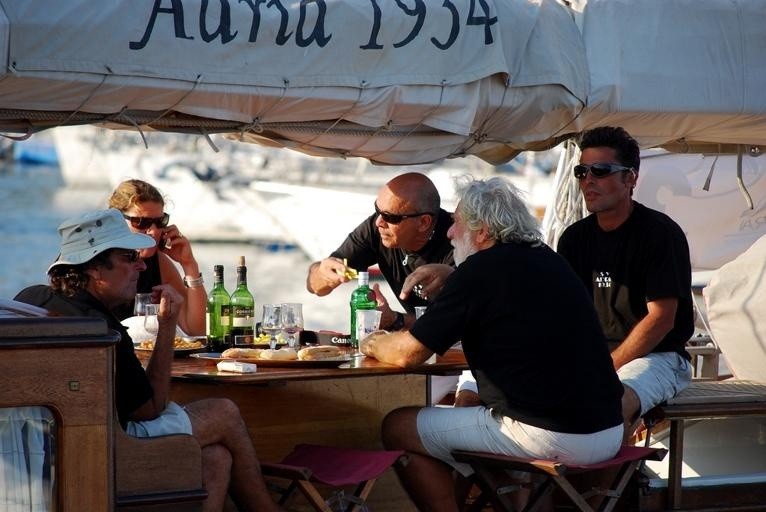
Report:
45,207,156,275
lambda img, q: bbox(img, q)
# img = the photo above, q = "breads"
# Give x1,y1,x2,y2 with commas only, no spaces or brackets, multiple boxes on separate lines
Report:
221,345,345,362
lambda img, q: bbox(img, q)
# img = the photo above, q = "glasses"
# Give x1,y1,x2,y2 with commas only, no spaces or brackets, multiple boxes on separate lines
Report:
374,201,434,224
121,213,170,229
113,251,140,263
573,162,633,180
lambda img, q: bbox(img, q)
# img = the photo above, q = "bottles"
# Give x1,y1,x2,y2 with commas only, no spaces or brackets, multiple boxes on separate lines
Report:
228,255,255,348
349,271,377,349
206,265,232,353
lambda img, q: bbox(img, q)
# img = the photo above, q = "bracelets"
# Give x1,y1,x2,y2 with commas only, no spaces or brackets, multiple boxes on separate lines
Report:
389,313,404,332
183,274,203,289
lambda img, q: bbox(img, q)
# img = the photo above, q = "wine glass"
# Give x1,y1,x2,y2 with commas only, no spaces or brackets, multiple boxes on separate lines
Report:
280,303,304,348
260,302,282,350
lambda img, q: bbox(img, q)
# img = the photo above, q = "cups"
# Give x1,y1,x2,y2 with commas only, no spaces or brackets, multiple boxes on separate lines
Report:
132,291,160,340
355,308,383,353
414,305,427,321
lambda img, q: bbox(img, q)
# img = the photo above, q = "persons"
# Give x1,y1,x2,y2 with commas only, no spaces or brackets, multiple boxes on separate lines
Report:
13,208,278,512
557,126,693,421
108,179,209,336
360,174,625,509
306,172,479,407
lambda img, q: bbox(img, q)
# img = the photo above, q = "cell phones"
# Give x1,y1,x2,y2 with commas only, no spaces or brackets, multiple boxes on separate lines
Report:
158,231,167,251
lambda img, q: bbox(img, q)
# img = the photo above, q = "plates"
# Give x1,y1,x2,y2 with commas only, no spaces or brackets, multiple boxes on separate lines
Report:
133,343,208,356
190,352,351,368
253,344,288,349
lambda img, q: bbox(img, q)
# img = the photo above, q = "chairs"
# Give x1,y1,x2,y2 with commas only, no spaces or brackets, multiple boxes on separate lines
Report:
0,298,208,512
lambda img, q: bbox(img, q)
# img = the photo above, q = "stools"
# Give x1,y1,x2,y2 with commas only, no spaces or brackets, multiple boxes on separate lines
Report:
256,439,409,512
633,382,765,511
448,442,671,510
